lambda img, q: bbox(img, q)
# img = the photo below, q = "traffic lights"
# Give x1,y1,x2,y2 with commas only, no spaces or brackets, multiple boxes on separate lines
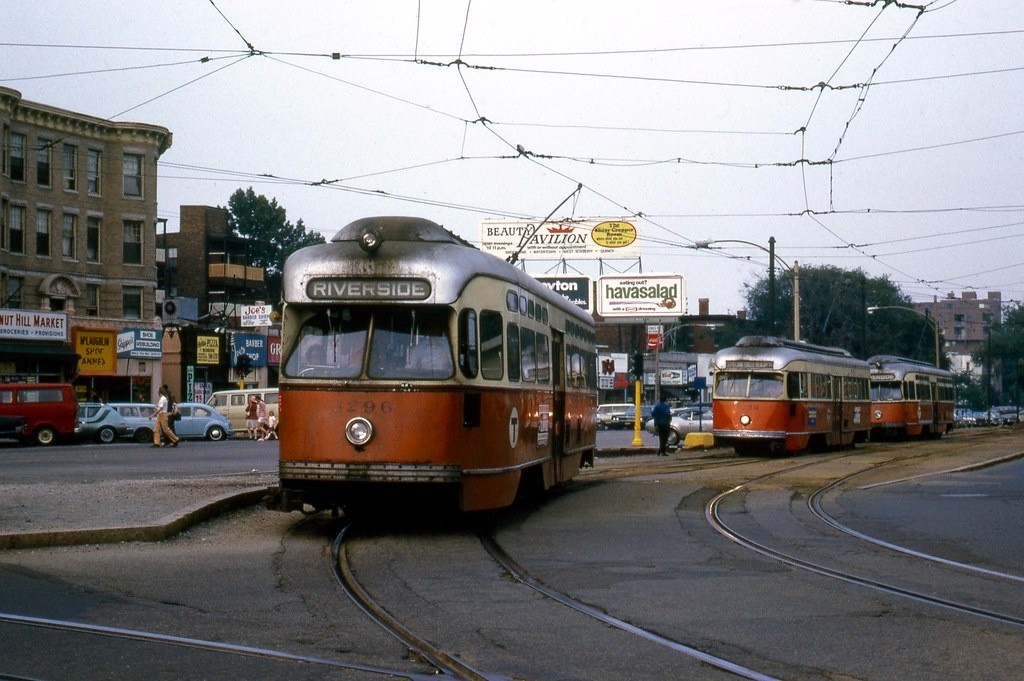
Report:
244,355,254,375
631,353,644,376
237,355,245,376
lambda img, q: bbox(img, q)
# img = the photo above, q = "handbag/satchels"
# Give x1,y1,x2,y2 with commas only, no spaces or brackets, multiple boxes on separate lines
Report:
245,403,251,417
172,405,181,421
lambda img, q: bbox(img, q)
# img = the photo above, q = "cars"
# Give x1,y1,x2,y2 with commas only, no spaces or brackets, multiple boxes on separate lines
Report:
171,402,234,442
74,401,130,444
953,404,1024,426
105,402,159,443
597,401,714,445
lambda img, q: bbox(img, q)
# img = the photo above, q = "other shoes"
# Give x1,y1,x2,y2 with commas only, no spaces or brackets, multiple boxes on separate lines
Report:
265,433,271,440
661,453,669,457
170,440,180,447
257,438,264,442
150,444,160,448
656,450,660,457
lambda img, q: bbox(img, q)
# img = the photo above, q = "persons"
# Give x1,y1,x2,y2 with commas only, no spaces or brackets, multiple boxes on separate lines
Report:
652,394,672,456
305,345,331,377
244,394,279,443
148,384,180,448
407,325,453,370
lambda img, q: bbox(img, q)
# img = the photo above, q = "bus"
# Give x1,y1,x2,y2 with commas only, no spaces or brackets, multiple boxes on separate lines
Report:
858,310,956,443
276,182,598,514
709,285,873,457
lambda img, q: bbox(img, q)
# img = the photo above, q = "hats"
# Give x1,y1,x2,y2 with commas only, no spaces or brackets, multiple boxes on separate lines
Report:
250,398,257,404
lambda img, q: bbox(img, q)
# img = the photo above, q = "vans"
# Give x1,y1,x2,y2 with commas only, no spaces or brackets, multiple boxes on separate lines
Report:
195,387,279,439
1,382,80,446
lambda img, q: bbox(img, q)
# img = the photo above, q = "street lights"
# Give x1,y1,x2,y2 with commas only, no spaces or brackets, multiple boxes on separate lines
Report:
653,317,724,406
867,294,942,369
694,238,801,343
